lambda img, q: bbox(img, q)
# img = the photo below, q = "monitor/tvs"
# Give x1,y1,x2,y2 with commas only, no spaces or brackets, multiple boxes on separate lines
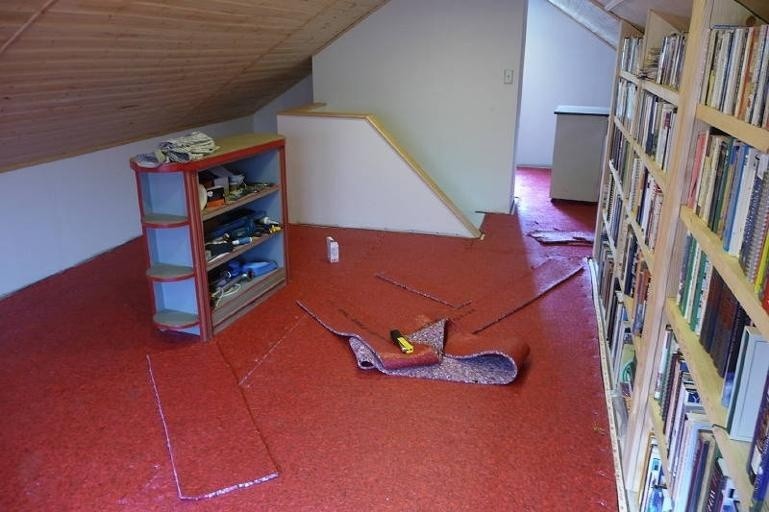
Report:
689,133,769,317
621,35,643,75
614,77,638,132
635,434,674,511
675,229,769,512
628,157,663,247
654,324,745,511
702,24,769,130
603,173,623,246
642,30,687,92
632,90,678,175
610,124,631,191
620,228,651,337
596,231,612,317
605,277,636,394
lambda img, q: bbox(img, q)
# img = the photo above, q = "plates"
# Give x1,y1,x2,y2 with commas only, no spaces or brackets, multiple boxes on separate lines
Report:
588,1,769,512
549,105,609,202
129,138,293,341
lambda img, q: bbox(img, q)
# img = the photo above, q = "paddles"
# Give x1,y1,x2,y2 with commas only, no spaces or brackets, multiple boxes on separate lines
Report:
295,283,525,395
0,166,620,511
445,259,583,332
373,270,471,308
146,341,278,500
525,230,593,246
